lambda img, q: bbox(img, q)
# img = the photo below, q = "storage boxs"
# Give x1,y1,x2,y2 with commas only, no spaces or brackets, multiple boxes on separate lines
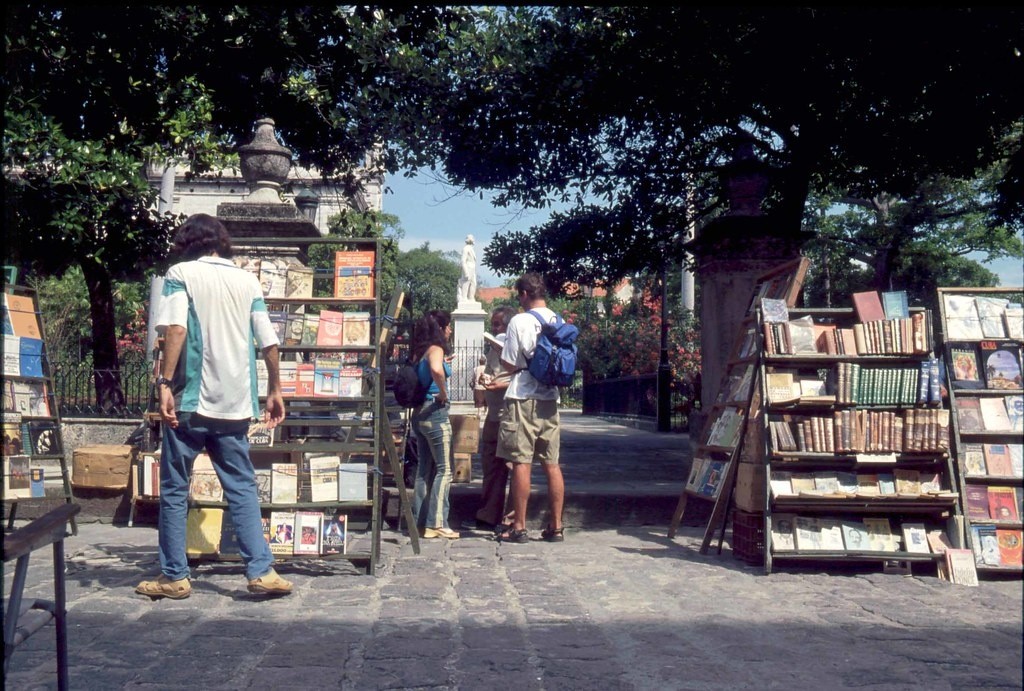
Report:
71,443,135,490
451,453,472,483
447,413,480,454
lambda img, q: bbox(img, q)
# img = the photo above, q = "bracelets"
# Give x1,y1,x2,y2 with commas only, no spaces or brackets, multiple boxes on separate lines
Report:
157,377,173,386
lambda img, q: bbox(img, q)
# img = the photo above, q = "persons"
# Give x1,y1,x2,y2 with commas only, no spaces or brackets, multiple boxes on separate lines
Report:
260,259,289,297
270,322,279,336
411,309,459,538
499,272,566,543
132,213,349,604
480,307,519,531
474,355,488,410
461,234,477,302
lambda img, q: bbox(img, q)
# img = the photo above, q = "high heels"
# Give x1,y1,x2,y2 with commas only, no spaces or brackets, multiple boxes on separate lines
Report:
424,527,460,539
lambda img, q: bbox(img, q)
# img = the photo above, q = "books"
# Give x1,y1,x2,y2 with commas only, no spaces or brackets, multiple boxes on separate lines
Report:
902,523,1023,586
253,309,373,346
687,457,729,499
966,485,1024,520
482,331,504,348
769,409,950,452
707,407,746,447
189,452,371,501
187,508,347,557
770,467,960,501
246,404,278,447
961,443,1024,476
735,272,934,357
229,256,313,297
951,348,1024,389
772,513,894,551
0,294,61,497
716,365,755,401
256,359,365,396
766,358,941,404
955,395,1024,431
335,251,374,299
944,294,1024,339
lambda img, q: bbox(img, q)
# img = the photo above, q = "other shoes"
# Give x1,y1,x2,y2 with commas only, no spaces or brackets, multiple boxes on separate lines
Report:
461,519,496,530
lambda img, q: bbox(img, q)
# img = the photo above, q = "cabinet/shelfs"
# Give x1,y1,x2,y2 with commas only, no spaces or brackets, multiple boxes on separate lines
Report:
752,307,965,576
669,256,808,553
936,284,1023,574
179,238,386,575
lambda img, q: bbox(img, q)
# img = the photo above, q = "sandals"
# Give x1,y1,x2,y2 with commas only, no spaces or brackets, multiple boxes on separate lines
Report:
135,573,192,599
495,526,528,543
541,523,564,542
246,568,294,593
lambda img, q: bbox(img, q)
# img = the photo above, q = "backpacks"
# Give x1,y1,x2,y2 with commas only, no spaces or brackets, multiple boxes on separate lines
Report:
522,310,580,386
393,345,433,408
